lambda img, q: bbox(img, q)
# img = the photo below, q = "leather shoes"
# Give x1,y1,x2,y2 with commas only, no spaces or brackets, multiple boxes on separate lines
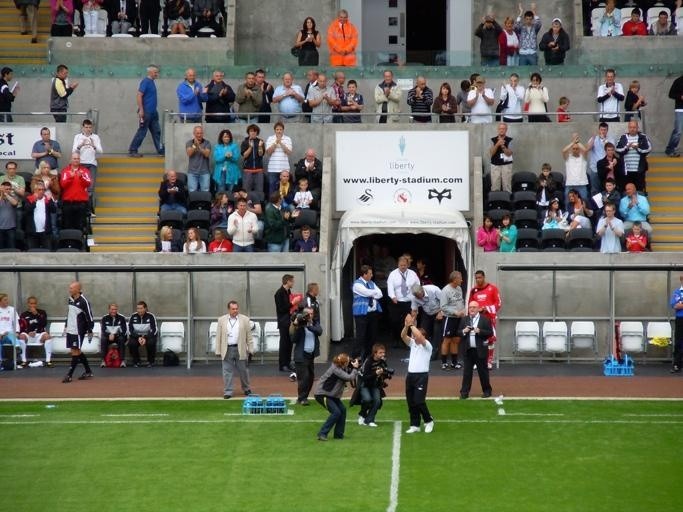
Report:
244,389,251,396
224,395,231,399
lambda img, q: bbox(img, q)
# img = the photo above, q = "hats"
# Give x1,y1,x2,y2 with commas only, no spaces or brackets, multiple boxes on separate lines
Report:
476,76,485,83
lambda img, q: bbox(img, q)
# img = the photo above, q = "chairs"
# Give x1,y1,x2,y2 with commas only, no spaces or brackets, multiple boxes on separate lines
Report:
156,156,321,252
513,318,675,368
0,161,99,252
1,316,285,367
481,167,609,252
77,0,224,37
590,8,683,36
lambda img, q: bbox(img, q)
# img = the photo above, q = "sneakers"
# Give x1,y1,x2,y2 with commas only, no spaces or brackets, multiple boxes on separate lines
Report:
128,151,143,158
16,361,156,383
666,152,680,157
279,363,493,441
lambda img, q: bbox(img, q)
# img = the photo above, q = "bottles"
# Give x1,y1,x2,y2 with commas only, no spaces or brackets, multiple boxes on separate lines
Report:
246,397,284,414
497,395,505,415
605,356,632,374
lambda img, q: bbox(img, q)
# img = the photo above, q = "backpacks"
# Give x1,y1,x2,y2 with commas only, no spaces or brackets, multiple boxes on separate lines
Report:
163,348,180,366
104,348,120,368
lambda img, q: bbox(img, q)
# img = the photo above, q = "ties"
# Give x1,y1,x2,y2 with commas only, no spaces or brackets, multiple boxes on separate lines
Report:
366,282,373,308
401,272,407,297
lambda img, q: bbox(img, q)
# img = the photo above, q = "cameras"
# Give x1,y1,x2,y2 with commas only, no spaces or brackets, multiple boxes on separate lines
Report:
529,85,538,89
467,326,475,331
348,358,363,369
382,367,395,379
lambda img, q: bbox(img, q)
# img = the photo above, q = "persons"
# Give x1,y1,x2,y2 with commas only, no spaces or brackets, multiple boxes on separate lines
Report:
0,67,20,122
49,65,80,122
349,342,389,428
326,8,359,68
17,296,56,368
311,352,360,442
669,274,683,373
50,0,227,37
60,281,95,384
274,274,323,406
0,119,105,249
294,17,321,66
13,0,41,43
589,0,683,37
352,254,502,400
472,2,571,64
100,302,129,368
216,300,255,399
664,75,683,158
0,294,22,371
400,308,435,433
130,301,158,368
129,66,654,254
